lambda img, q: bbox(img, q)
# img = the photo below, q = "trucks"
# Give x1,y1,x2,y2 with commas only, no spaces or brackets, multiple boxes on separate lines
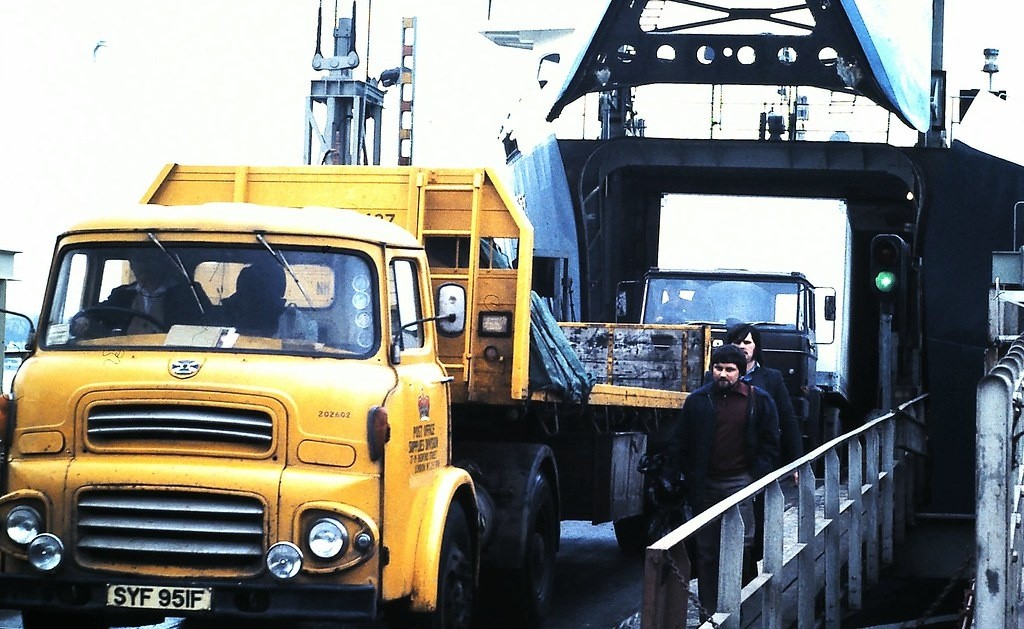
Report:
614,264,848,469
1,162,712,629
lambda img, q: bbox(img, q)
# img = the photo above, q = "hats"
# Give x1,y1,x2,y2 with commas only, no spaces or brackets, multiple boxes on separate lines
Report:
711,345,746,376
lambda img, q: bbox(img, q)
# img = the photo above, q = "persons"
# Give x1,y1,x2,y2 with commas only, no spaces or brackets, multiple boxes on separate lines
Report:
69,243,219,338
659,341,782,629
699,323,804,565
